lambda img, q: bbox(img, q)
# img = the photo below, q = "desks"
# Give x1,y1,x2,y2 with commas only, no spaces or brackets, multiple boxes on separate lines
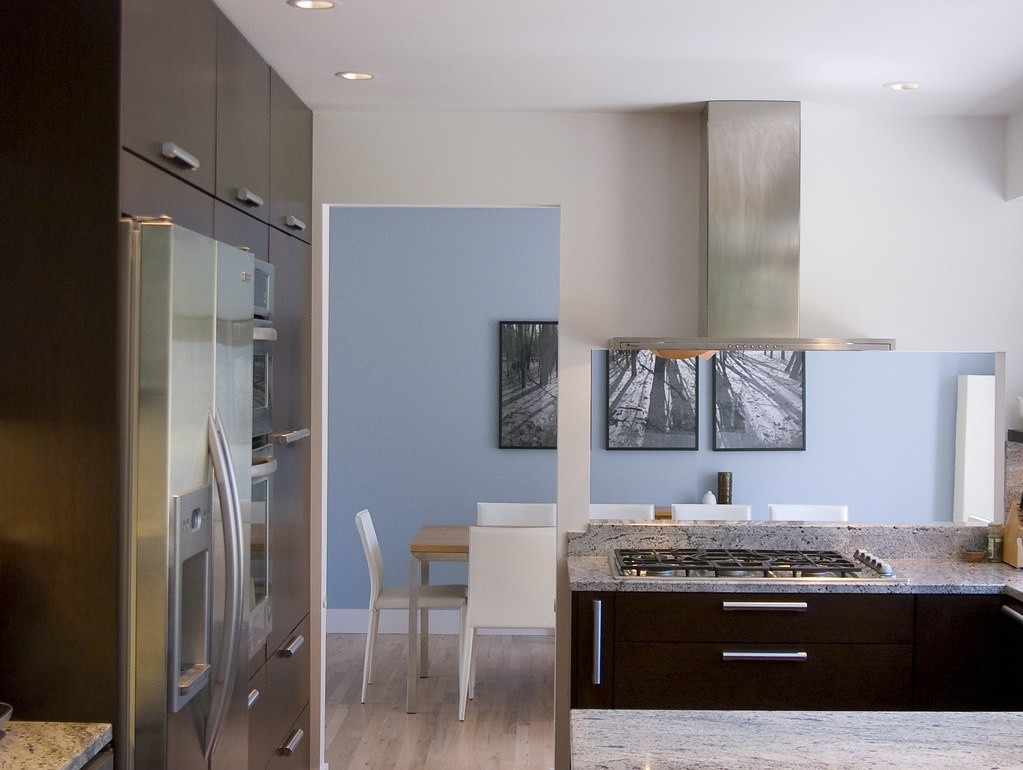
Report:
406,525,469,714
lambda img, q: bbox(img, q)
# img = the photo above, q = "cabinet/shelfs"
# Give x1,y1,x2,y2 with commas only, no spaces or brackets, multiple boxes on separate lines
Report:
0,0,313,242
570,590,1023,710
268,225,314,770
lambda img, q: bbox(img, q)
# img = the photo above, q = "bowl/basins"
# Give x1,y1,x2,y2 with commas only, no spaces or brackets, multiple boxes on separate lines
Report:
963,547,986,561
704,491,716,505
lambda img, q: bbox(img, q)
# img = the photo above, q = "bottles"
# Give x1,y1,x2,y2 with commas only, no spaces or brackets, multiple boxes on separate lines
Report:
989,523,1003,562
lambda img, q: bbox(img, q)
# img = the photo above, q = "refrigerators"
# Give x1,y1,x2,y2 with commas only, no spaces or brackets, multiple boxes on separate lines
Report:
117,215,255,770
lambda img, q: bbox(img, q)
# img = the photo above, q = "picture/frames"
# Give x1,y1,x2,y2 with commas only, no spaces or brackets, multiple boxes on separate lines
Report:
607,350,699,450
499,321,558,449
712,349,806,451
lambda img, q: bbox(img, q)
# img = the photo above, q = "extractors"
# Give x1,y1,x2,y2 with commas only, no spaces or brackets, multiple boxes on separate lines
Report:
612,101,896,351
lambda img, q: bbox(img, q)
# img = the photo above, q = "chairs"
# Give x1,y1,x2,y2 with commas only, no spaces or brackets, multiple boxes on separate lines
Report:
356,503,556,722
590,503,848,524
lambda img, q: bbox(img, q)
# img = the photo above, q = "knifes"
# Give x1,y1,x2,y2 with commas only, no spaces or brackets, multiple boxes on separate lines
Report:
1019,491,1023,510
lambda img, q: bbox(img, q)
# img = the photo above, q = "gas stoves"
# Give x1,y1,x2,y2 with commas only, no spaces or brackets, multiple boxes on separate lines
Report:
607,548,910,581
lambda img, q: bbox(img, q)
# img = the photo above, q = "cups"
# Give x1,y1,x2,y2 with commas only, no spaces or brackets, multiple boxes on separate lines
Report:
717,472,733,504
